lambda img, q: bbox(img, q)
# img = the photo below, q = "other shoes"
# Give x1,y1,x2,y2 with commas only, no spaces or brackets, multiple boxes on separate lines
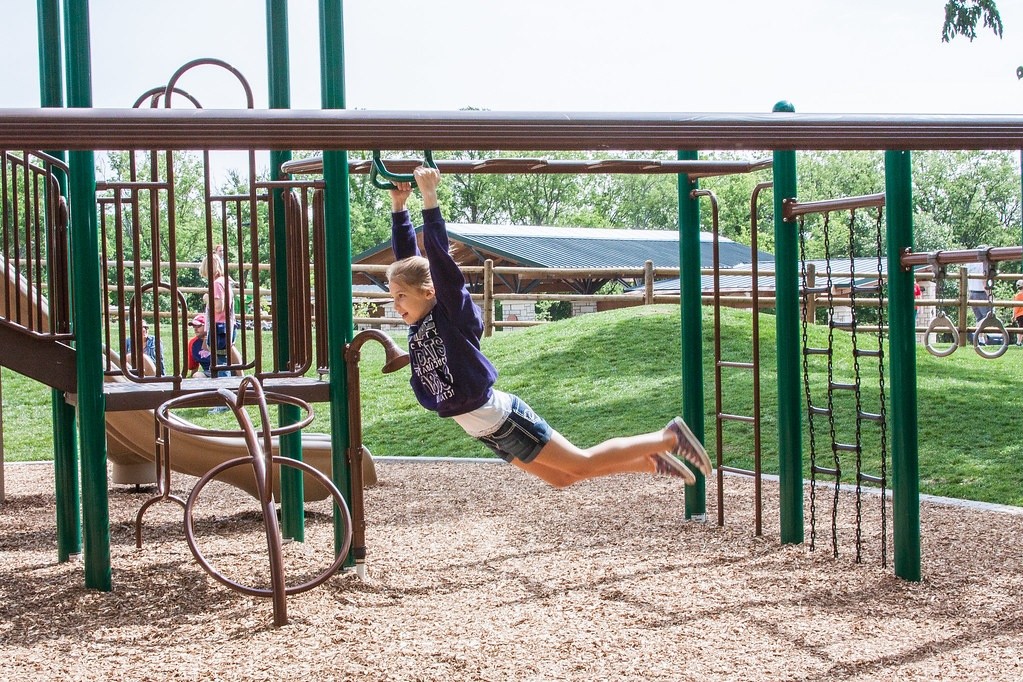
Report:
1016,343,1021,346
979,342,985,346
209,407,229,415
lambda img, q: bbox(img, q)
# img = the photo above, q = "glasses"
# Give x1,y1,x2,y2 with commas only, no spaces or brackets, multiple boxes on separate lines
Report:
142,325,149,330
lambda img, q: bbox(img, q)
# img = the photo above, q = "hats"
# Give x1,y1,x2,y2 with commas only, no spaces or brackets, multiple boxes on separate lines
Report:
1016,280,1023,289
188,314,206,327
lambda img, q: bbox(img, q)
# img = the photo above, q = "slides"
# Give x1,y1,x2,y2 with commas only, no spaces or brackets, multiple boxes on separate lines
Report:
0,253,378,503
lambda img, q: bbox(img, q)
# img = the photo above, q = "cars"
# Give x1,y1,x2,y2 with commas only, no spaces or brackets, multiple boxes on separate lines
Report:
968,332,1005,345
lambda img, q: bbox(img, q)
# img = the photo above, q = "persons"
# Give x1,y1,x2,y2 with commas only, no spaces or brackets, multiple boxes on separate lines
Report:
1011,279,1023,346
913,274,921,316
964,246,992,344
197,244,277,413
124,319,164,377
186,315,237,377
385,164,713,488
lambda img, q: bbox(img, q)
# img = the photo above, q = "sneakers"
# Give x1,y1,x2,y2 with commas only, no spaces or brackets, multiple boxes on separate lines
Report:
648,450,696,486
666,416,713,477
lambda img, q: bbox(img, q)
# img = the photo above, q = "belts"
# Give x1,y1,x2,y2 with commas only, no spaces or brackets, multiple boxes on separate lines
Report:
971,290,985,294
